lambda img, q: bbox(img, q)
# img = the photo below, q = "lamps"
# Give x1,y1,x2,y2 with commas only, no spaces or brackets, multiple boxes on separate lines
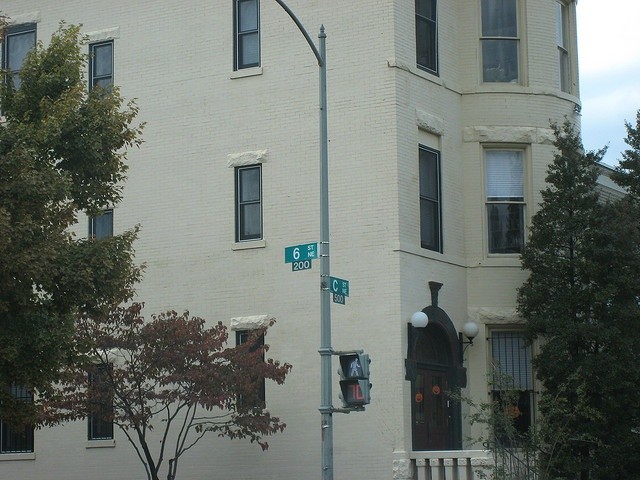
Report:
459,319,480,388
403,312,426,379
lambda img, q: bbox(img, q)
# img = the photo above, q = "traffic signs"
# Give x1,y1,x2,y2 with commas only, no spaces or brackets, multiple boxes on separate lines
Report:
330,276,349,304
284,242,317,271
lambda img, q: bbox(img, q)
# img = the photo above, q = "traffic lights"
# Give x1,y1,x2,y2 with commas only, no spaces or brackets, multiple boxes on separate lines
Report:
338,354,373,405
